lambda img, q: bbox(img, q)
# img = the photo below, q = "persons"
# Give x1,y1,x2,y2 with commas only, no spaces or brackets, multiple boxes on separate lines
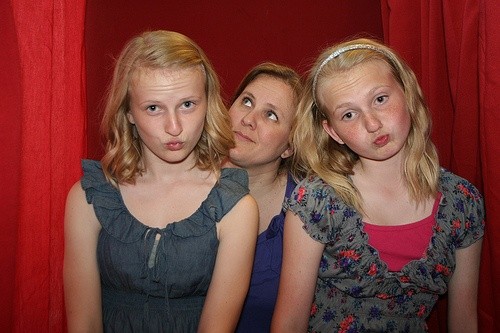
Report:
221,62,304,332
62,30,258,333
270,38,485,333
